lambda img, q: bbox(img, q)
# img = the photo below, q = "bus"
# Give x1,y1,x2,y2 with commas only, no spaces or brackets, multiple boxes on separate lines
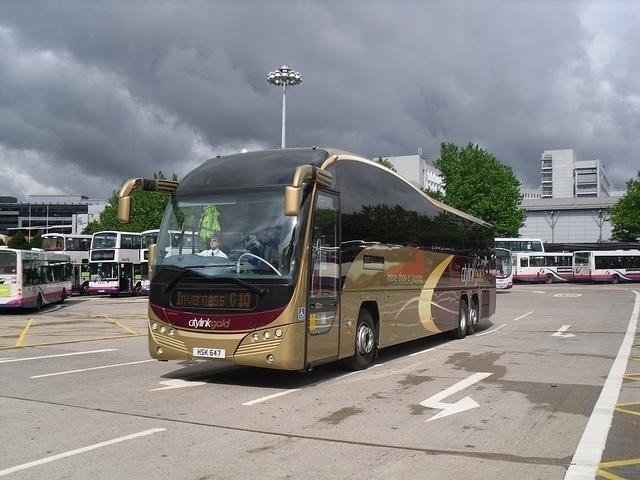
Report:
0,228,203,311
493,235,640,289
115,148,496,375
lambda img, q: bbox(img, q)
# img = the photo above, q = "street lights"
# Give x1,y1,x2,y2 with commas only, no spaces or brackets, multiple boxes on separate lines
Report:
266,65,301,149
545,210,560,242
592,210,608,242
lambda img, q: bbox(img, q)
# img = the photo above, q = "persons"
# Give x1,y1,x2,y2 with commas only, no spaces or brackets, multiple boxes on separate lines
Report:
194,236,228,259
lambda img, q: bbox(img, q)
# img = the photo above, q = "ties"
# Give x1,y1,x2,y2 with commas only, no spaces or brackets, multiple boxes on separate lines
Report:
212,252,215,257
